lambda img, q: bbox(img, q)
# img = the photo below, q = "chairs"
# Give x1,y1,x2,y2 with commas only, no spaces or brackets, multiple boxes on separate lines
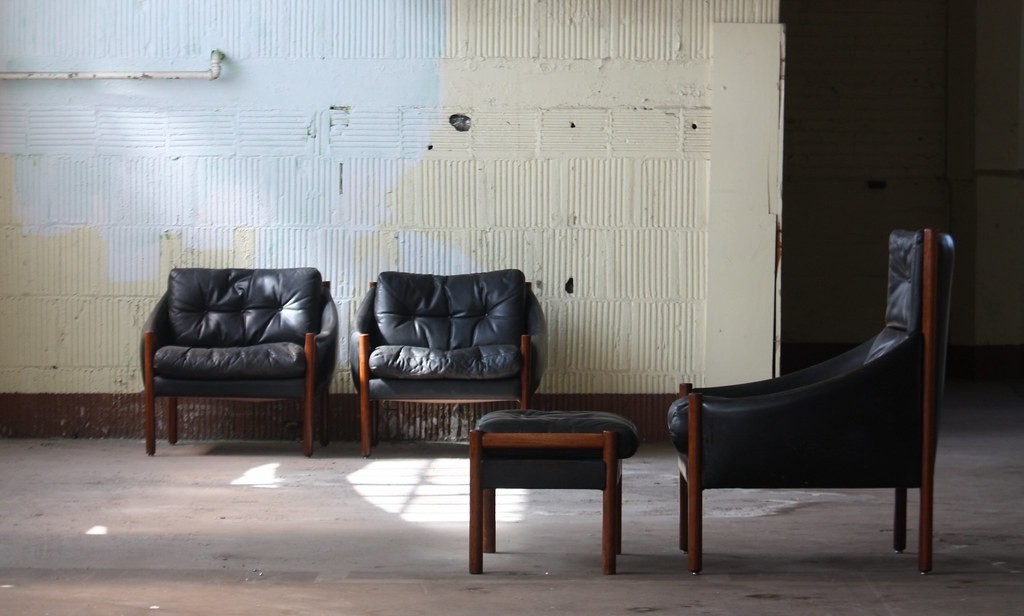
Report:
348,270,548,458
140,267,338,458
666,228,956,576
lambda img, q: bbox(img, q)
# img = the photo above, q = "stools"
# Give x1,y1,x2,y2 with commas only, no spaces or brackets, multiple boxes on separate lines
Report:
468,409,639,573
